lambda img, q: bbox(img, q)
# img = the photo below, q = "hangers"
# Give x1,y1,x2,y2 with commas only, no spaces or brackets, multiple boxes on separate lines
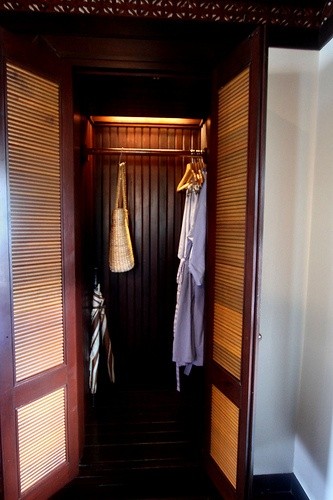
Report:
175,150,209,194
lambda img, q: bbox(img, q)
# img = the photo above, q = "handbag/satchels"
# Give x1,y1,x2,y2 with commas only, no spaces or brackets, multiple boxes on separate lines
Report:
109,162,134,273
